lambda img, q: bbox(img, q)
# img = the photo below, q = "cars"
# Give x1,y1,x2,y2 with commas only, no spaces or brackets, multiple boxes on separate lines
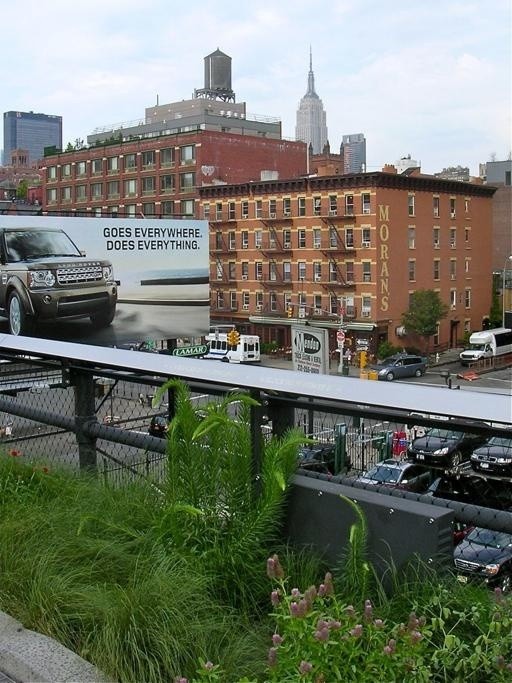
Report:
453,526,512,592
297,443,352,474
354,418,511,542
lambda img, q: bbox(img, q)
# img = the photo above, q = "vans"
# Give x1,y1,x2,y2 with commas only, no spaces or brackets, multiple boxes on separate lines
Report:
459,328,512,366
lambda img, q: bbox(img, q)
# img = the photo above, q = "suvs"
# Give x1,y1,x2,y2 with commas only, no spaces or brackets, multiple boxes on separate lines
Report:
370,355,426,381
148,410,208,439
1,227,119,336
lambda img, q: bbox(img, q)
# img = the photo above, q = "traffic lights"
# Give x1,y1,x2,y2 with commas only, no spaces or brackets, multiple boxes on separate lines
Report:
288,307,293,317
227,331,240,346
360,351,367,368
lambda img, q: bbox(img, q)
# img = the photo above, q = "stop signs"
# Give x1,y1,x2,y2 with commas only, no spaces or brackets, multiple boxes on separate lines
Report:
336,332,345,343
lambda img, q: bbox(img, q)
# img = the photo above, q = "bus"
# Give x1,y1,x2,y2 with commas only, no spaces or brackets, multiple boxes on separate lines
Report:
204,332,261,364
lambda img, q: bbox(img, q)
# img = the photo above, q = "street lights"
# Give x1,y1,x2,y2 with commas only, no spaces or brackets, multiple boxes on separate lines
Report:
502,255,511,327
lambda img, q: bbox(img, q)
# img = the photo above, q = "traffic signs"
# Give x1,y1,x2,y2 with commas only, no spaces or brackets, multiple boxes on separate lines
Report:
299,308,306,319
